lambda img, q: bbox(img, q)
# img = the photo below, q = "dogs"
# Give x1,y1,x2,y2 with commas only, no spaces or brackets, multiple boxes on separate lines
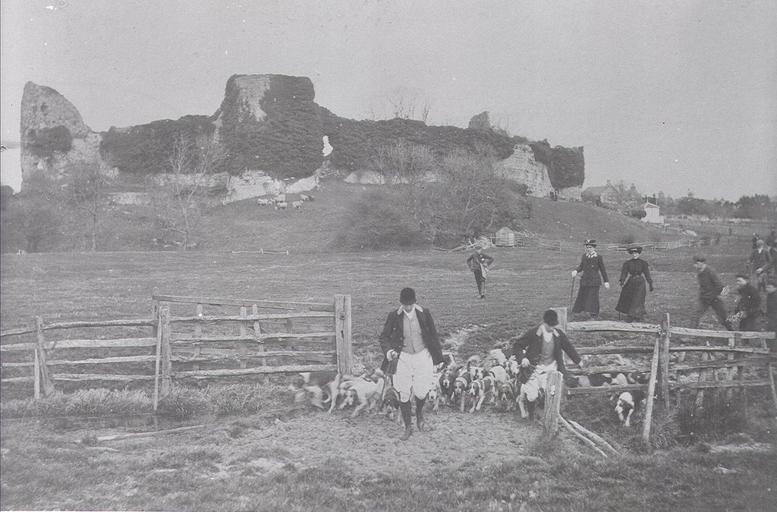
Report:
280,350,648,428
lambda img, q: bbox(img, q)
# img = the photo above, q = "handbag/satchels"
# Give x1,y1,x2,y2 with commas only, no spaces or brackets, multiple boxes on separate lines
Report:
480,264,489,280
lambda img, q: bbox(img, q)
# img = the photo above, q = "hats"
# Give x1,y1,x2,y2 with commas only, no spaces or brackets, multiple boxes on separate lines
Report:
399,286,417,306
542,309,559,326
582,239,597,246
627,246,642,255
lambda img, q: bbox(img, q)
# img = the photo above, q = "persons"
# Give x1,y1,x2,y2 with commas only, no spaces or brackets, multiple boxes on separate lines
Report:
466,243,495,297
692,230,776,331
379,288,443,438
572,237,653,322
515,308,581,424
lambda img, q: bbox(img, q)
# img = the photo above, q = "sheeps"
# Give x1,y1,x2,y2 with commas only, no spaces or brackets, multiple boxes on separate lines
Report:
256,193,315,210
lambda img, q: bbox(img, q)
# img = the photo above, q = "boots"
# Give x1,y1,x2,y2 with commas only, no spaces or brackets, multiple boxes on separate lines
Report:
414,392,428,430
399,399,414,440
526,397,536,423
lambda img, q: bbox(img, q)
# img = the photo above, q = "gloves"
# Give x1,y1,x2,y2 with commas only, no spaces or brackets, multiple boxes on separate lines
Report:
649,282,654,292
